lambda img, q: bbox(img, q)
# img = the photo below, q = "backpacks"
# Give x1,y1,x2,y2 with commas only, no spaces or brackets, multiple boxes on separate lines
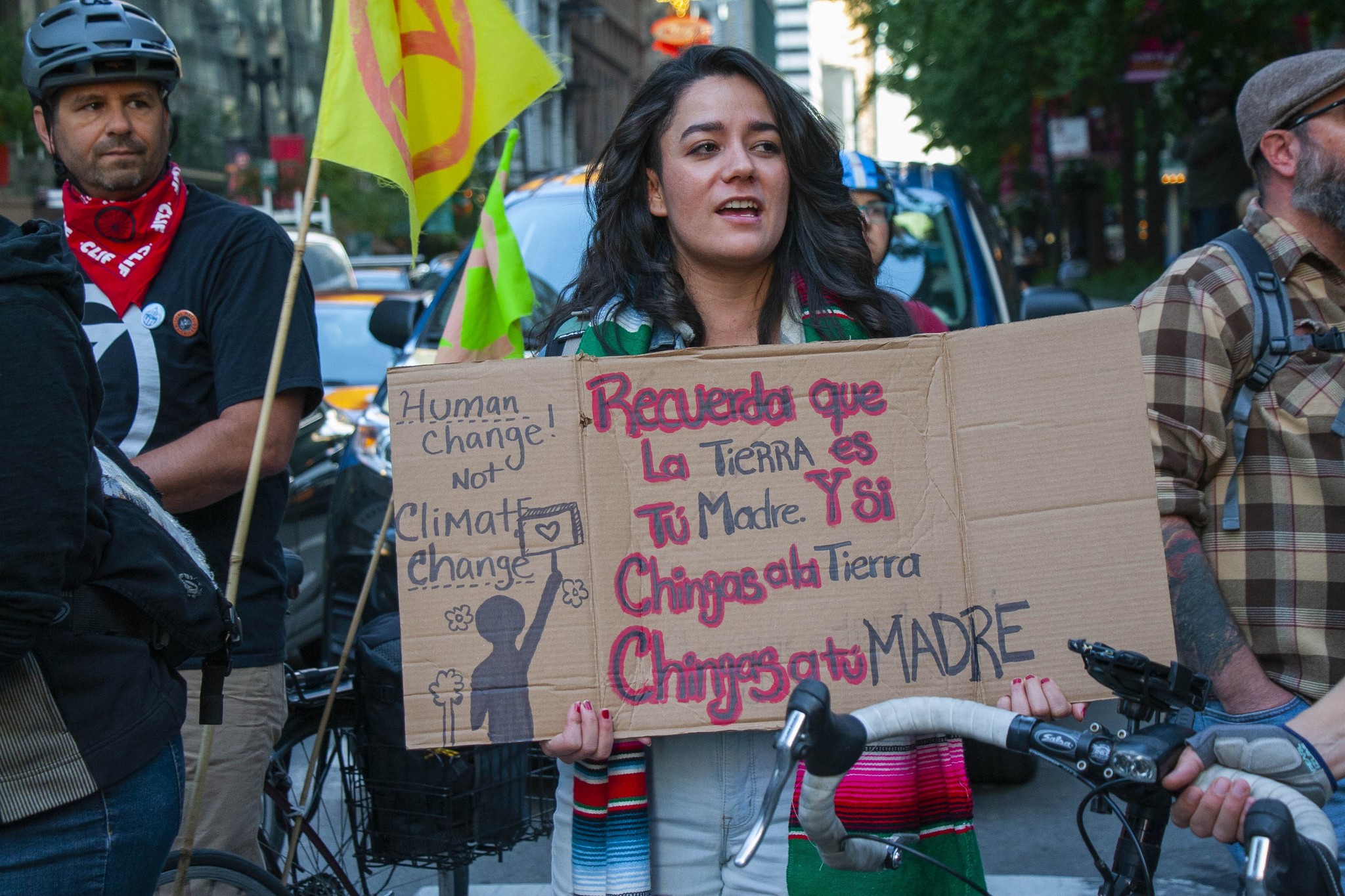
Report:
341,608,534,866
86,424,243,725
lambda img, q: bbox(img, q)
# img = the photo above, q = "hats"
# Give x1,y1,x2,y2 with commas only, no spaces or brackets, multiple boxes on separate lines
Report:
1235,49,1345,167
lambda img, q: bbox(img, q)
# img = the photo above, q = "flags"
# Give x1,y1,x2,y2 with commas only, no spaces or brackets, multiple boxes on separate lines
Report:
307,0,565,278
434,128,539,370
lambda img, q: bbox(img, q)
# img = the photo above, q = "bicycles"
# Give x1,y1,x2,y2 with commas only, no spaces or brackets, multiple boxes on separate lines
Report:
252,548,560,896
151,848,295,896
734,638,1340,896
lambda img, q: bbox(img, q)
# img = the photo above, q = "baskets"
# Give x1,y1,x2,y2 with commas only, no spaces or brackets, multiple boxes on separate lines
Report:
322,669,563,877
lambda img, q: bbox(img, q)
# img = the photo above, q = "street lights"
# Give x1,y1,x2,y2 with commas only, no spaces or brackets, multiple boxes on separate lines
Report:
236,32,284,160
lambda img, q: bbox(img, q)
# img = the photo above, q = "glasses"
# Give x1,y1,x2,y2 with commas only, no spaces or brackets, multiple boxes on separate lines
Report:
850,199,898,224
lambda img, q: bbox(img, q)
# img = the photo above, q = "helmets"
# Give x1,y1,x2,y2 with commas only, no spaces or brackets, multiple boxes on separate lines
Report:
21,0,184,113
839,151,897,215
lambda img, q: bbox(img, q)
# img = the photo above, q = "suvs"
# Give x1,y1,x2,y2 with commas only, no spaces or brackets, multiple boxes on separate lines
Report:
321,160,1089,674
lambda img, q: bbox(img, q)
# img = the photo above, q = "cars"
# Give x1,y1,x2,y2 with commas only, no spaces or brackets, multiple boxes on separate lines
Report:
272,224,462,652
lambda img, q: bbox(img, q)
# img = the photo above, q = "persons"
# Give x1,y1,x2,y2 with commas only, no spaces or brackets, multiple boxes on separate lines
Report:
1156,674,1345,851
1127,43,1343,895
12,0,327,896
520,36,1092,892
835,146,956,339
0,213,190,895
